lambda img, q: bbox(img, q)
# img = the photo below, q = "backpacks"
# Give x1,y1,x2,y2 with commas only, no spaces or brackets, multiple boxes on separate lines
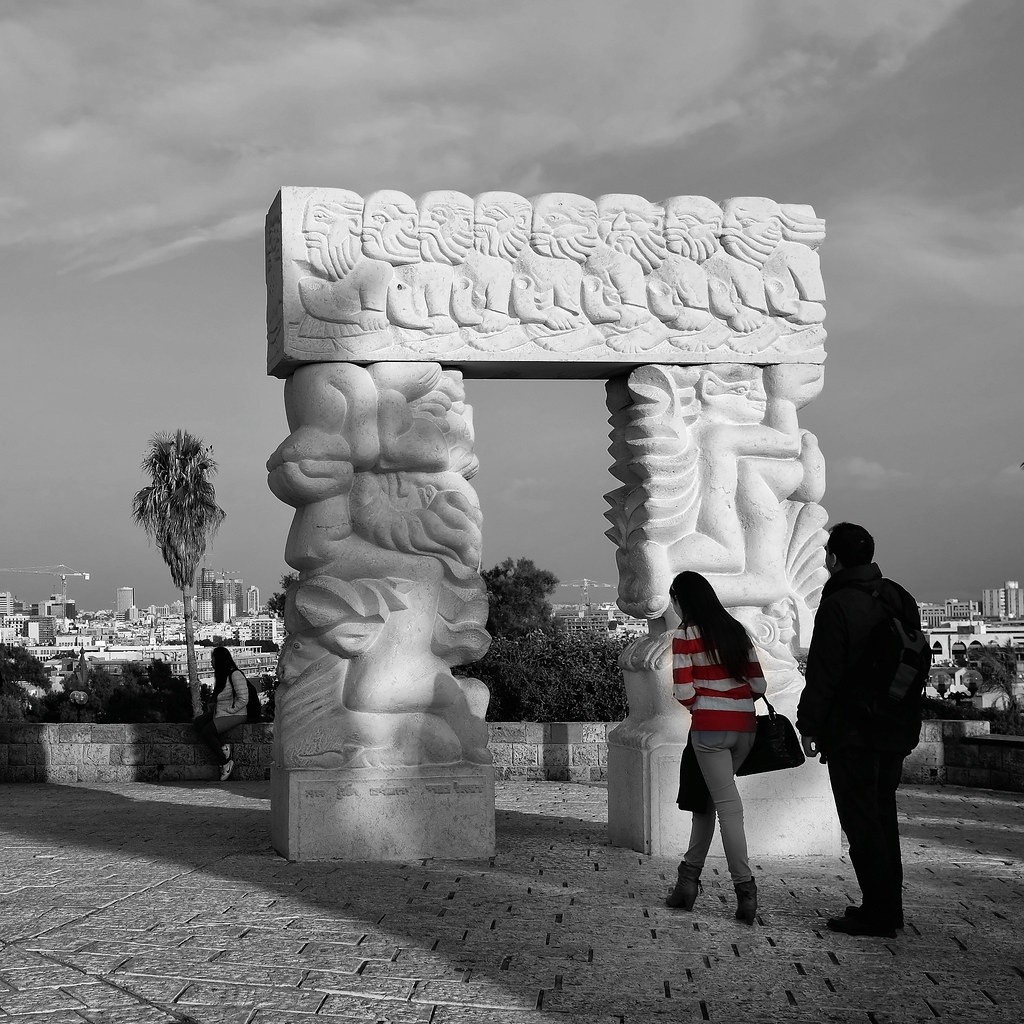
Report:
840,579,933,729
228,668,261,724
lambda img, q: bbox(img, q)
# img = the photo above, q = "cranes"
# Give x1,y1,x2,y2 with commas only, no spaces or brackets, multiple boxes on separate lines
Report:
560,577,617,603
216,568,240,579
0,564,90,618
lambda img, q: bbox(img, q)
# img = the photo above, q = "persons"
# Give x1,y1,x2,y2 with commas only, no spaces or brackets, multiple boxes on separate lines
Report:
663,571,766,926
192,647,249,780
795,520,930,938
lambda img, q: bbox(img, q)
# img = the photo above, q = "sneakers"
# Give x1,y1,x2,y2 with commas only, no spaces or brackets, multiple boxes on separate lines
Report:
222,743,231,760
217,760,235,781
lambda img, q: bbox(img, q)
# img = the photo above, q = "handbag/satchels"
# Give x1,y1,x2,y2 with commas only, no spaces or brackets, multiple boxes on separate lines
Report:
735,694,806,777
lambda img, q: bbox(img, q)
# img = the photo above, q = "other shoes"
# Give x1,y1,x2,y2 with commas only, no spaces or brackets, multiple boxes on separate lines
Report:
827,905,904,939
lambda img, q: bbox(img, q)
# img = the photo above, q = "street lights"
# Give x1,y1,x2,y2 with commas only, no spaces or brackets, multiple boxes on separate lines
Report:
70,690,89,723
931,670,983,706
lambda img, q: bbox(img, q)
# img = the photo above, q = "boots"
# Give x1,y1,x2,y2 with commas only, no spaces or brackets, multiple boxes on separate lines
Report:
733,875,758,926
666,861,704,911
